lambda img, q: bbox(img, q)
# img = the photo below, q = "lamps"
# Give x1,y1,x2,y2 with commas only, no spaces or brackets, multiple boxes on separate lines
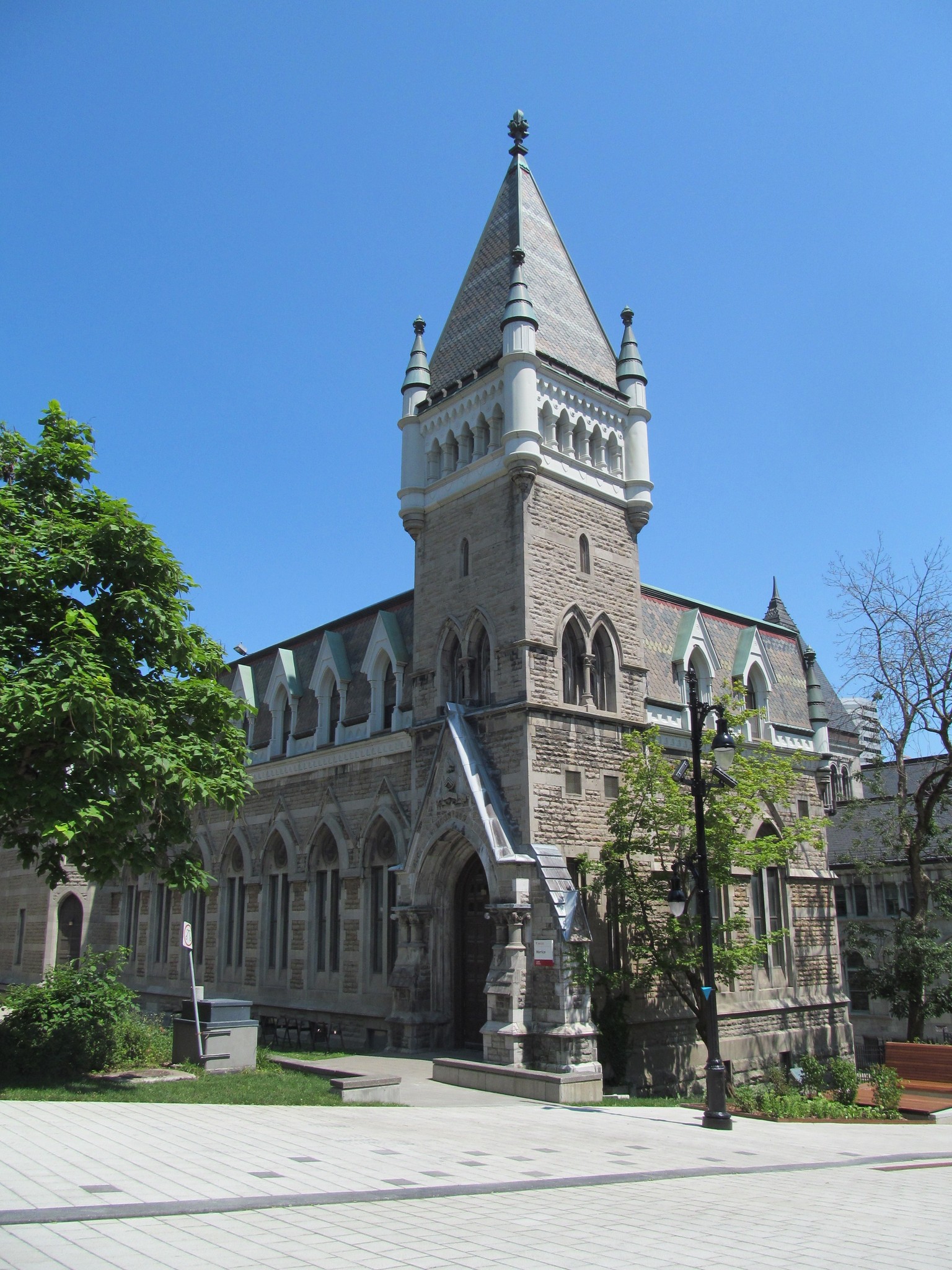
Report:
317,1027,329,1037
233,641,249,656
331,1029,341,1036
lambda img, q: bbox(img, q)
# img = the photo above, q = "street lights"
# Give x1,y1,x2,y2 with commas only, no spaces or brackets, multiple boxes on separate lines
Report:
671,666,738,1129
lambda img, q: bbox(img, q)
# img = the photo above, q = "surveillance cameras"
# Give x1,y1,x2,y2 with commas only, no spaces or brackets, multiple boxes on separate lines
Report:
710,765,738,788
671,759,690,782
